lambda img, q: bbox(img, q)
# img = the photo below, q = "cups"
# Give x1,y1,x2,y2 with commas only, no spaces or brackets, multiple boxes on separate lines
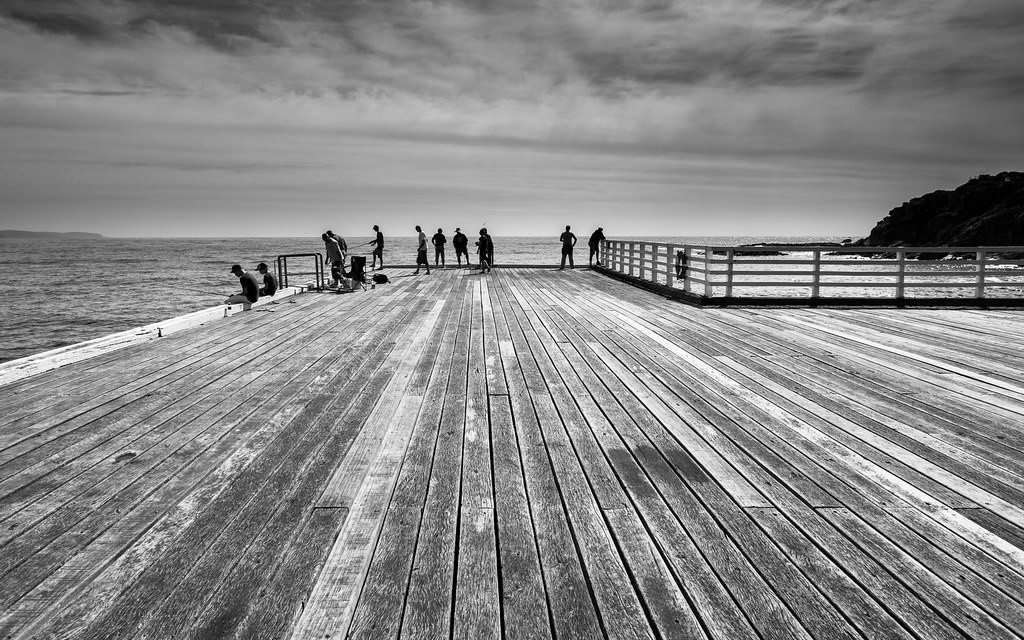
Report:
228,302,231,305
372,282,375,288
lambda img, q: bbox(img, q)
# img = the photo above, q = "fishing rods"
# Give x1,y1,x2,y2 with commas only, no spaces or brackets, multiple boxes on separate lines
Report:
257,282,337,291
347,242,370,249
323,262,331,273
162,284,233,298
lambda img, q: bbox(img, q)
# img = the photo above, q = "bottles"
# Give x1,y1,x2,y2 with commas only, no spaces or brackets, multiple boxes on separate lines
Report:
227,307,232,317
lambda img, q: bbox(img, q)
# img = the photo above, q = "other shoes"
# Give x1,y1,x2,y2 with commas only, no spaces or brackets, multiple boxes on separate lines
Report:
412,271,419,274
423,272,430,275
487,268,490,272
479,272,485,274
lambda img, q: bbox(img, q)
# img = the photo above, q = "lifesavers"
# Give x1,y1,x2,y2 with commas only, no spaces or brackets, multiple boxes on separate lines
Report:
676,251,687,279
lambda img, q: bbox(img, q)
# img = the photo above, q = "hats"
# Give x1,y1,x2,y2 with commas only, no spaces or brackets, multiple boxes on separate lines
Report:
255,263,267,271
372,225,378,229
230,265,242,273
454,227,462,232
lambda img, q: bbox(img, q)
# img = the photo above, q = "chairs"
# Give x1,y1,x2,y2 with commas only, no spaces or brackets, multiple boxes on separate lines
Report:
337,256,367,292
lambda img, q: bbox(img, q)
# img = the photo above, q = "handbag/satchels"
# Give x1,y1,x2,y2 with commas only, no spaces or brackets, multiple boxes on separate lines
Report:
373,274,391,283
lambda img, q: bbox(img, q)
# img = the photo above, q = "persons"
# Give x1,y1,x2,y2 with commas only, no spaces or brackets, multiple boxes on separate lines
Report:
453,227,471,268
321,233,346,288
368,225,384,270
588,227,606,270
560,225,577,270
475,227,493,274
225,265,259,304
413,226,431,274
431,228,446,268
326,230,347,266
255,263,278,297
